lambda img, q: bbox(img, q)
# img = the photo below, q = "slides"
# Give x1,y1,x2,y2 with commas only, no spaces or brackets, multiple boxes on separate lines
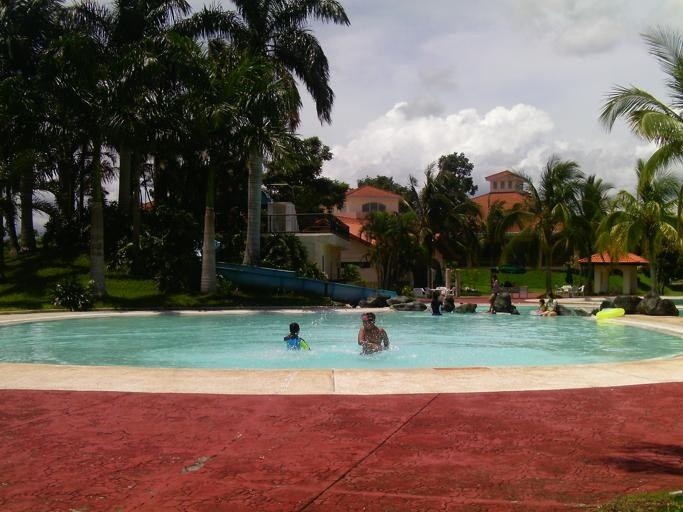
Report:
217,262,397,302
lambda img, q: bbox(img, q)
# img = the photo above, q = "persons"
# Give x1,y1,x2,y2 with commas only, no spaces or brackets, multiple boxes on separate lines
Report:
444,293,456,314
358,312,390,356
430,290,443,315
486,277,500,313
543,292,559,317
564,264,574,298
283,323,311,351
535,299,545,316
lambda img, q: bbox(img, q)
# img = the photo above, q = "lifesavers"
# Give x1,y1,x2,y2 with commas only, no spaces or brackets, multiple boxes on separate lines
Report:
596,307,625,320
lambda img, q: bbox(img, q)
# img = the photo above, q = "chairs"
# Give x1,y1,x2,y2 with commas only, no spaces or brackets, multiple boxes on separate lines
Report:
554,284,584,298
413,286,457,300
